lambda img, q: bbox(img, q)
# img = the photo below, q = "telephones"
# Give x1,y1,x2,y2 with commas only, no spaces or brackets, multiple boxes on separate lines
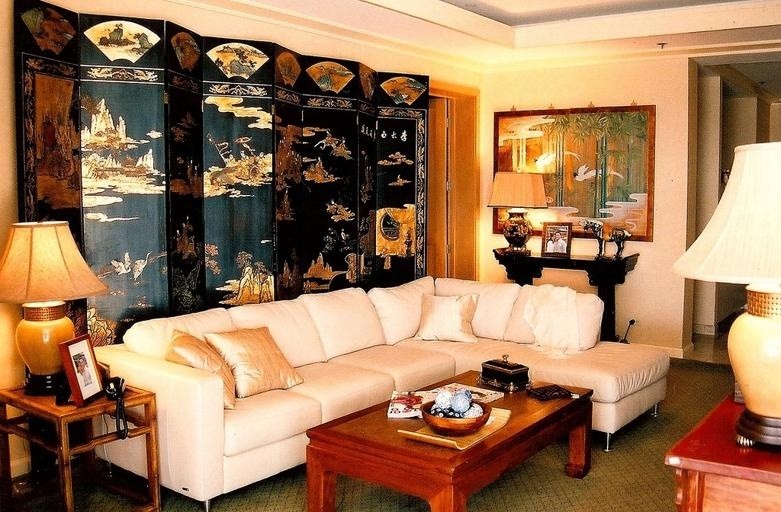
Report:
104,377,126,400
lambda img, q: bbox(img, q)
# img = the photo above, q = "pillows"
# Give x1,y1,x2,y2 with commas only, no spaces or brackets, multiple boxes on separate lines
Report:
228,299,327,367
203,326,304,399
123,307,233,360
300,287,385,360
417,292,480,343
507,285,604,352
367,276,435,346
166,329,237,409
435,278,520,340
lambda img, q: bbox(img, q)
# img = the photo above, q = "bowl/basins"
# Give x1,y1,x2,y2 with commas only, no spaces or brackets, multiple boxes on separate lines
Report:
420,401,492,435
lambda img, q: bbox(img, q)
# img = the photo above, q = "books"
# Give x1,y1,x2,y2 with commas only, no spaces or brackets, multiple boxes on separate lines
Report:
438,382,504,403
387,387,435,419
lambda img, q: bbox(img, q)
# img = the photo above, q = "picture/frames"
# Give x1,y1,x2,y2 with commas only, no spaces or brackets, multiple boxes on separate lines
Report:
60,335,107,406
541,222,572,258
493,105,656,242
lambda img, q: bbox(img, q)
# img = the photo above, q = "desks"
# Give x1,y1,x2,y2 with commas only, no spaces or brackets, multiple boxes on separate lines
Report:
1,381,161,511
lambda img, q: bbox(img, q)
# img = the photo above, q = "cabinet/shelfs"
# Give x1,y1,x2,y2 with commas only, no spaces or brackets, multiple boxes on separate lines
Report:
494,248,639,342
664,394,780,512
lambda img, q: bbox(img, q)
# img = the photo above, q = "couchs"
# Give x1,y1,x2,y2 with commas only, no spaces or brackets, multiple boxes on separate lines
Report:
94,276,670,512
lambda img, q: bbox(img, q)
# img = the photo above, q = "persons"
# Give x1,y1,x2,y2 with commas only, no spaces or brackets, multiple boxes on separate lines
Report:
470,390,485,400
76,357,91,384
545,232,566,251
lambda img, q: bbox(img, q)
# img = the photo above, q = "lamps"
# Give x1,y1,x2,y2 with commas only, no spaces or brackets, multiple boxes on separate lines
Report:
488,171,547,255
669,142,781,450
3,222,108,395
